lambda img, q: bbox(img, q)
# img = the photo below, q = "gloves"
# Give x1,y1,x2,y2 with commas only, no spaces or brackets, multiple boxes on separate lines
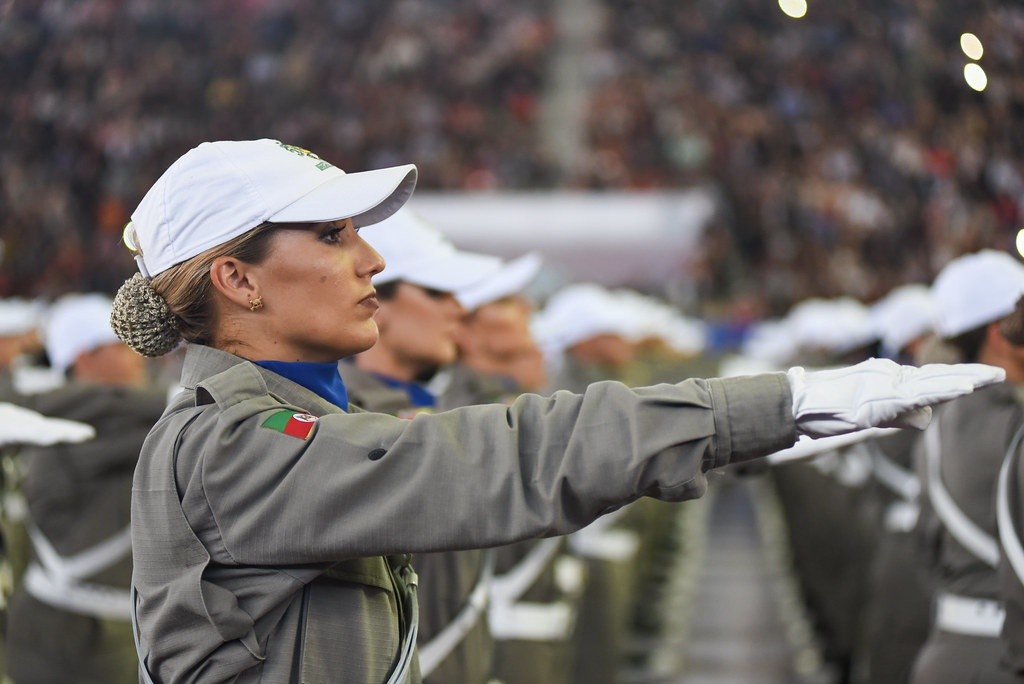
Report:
767,426,902,466
783,357,1006,440
0,403,96,447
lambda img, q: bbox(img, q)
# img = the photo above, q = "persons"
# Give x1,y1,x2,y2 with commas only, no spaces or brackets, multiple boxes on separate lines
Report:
0,0,1024,684
110,137,1006,682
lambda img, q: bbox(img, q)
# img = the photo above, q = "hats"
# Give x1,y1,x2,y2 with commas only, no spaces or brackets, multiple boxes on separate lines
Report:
355,210,485,291
545,280,708,357
455,249,542,316
135,137,418,278
43,290,128,369
742,234,1024,369
0,295,42,338
